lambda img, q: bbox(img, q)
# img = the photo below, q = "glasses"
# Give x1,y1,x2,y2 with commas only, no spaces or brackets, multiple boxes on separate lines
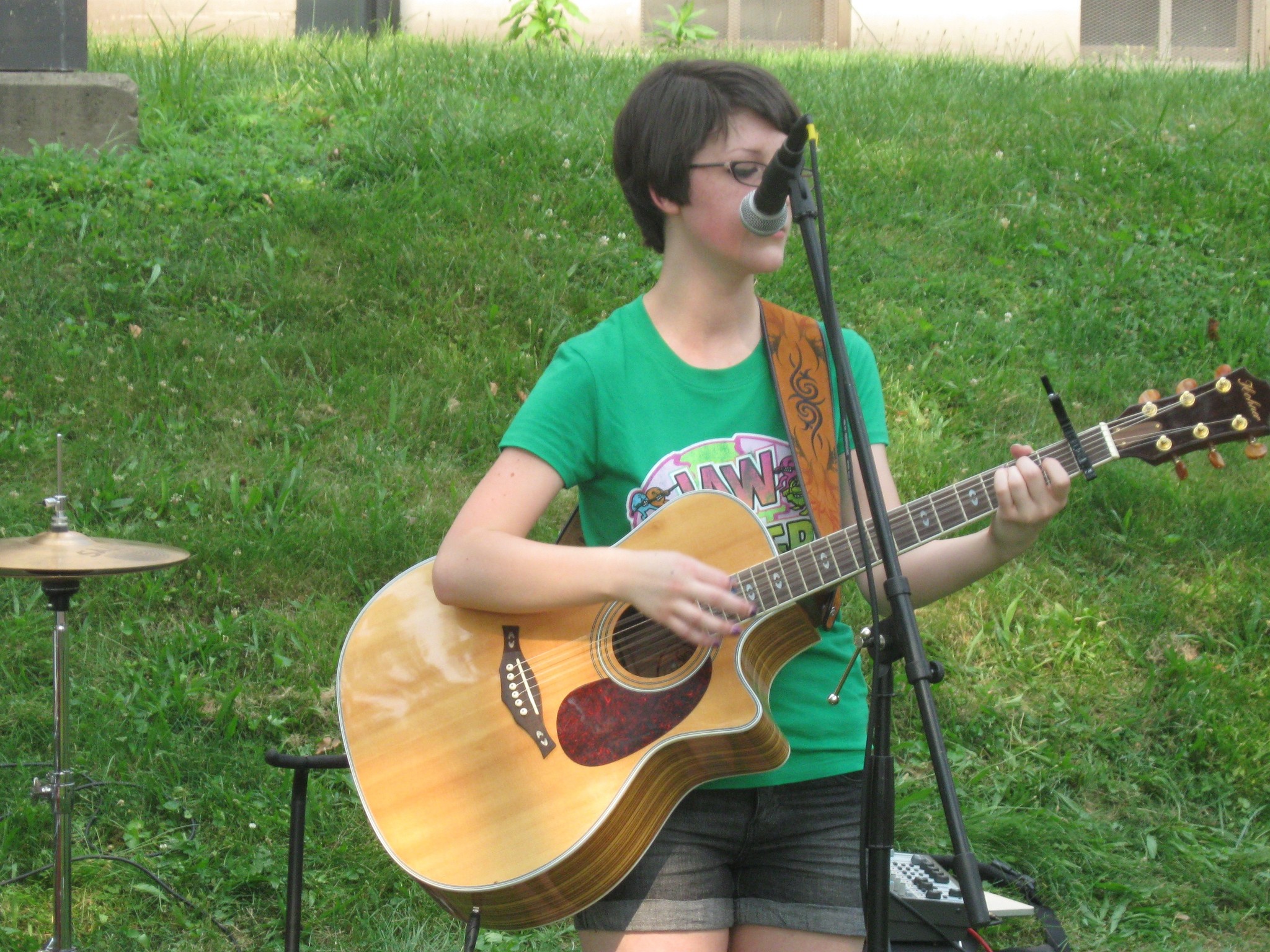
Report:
690,160,820,194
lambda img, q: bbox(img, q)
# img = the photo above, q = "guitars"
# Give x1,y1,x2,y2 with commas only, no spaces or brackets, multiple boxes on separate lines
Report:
328,357,1270,935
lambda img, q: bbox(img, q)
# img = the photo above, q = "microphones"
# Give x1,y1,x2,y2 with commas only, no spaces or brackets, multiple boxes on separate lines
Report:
739,112,815,237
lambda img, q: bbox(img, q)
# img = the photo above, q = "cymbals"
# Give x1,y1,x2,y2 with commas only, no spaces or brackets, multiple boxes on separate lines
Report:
0,530,197,576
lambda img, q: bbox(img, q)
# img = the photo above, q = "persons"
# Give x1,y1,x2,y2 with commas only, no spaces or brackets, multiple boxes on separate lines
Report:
426,66,1071,952
